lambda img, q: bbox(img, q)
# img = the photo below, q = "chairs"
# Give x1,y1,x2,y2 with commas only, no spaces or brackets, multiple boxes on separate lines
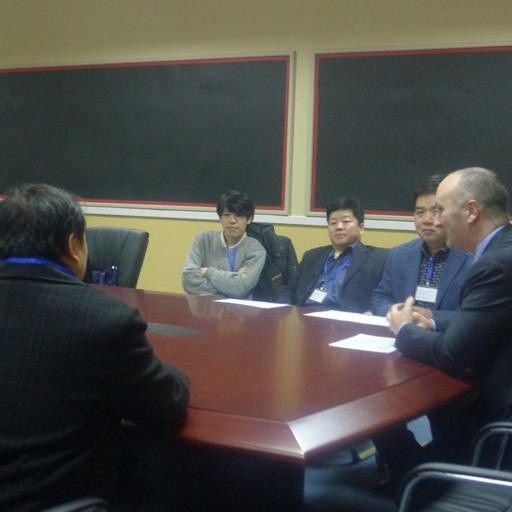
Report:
244,222,298,305
83,226,150,288
395,422,512,512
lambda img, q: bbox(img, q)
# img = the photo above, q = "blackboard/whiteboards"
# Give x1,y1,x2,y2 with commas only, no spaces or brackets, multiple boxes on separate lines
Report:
306,42,512,223
0,49,295,215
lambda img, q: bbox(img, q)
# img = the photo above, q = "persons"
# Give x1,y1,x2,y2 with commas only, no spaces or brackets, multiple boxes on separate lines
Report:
368,174,475,492
2,183,189,511
277,195,390,314
182,190,266,300
386,167,511,463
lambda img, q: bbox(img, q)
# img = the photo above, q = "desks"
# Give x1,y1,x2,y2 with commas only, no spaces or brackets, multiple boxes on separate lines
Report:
87,282,472,512
